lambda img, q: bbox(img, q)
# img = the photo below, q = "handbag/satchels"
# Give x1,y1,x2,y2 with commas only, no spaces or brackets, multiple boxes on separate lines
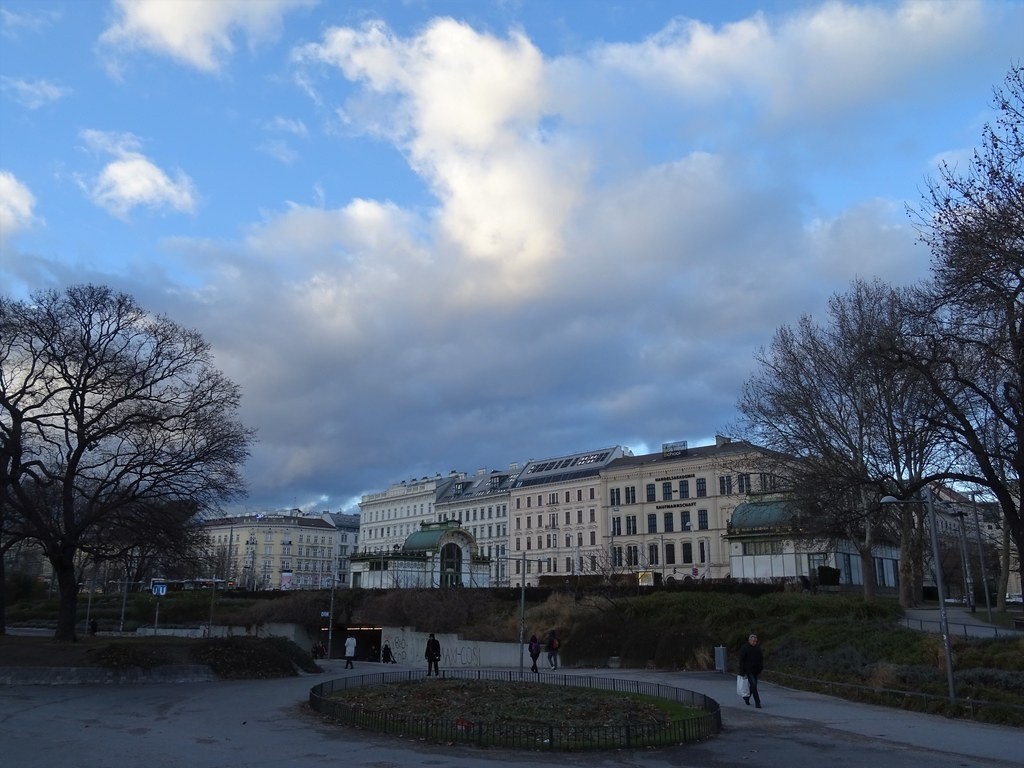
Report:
737,675,750,697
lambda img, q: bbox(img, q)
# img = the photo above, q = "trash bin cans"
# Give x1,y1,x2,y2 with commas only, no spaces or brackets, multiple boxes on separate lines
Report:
714,647,727,675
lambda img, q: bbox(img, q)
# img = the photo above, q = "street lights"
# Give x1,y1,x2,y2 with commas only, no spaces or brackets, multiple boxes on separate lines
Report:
498,552,549,678
879,488,957,708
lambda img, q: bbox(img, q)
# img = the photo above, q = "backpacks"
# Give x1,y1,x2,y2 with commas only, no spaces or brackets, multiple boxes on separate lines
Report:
531,640,540,655
551,637,560,650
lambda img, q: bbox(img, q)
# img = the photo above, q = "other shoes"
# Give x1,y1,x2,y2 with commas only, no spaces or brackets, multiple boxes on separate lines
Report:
551,666,555,670
755,703,761,708
743,697,750,705
553,670,557,672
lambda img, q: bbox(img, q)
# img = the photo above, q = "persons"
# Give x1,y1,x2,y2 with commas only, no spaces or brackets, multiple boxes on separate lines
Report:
738,634,764,708
367,644,378,662
311,638,328,659
424,633,441,677
528,635,540,673
342,632,356,669
381,644,392,664
543,629,560,672
